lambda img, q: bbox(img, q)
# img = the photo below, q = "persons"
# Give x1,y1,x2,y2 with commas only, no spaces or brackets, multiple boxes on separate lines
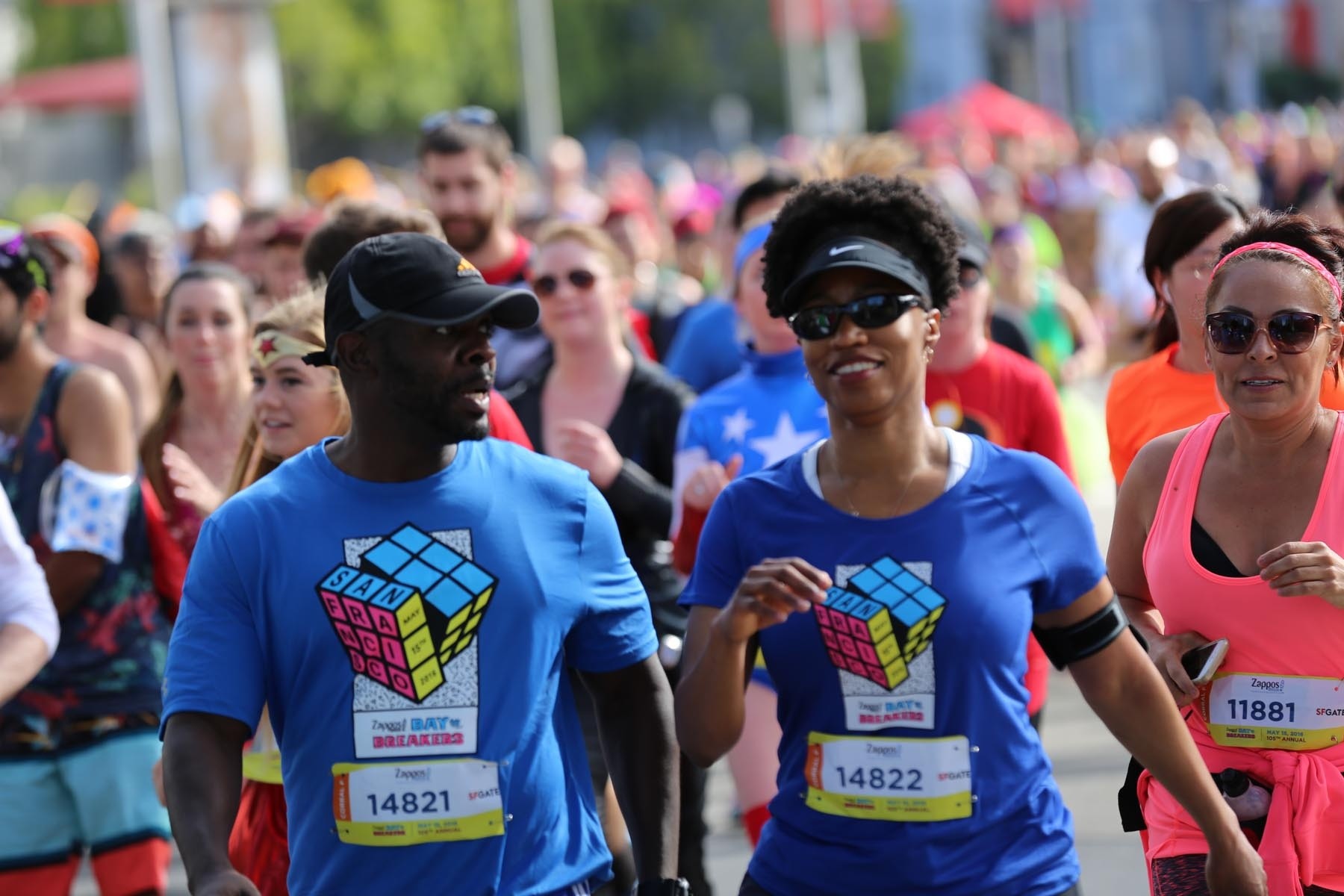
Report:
673,176,1267,895
158,233,688,895
1106,213,1344,896
0,98,1344,896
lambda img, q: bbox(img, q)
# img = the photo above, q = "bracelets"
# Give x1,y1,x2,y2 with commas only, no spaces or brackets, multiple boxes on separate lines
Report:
637,877,690,895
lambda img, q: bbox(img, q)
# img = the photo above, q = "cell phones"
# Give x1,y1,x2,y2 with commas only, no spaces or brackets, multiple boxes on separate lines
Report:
1182,640,1228,686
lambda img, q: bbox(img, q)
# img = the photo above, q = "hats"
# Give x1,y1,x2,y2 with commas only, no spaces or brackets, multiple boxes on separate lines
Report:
781,237,933,302
306,156,376,207
26,214,98,285
946,214,987,277
300,231,540,368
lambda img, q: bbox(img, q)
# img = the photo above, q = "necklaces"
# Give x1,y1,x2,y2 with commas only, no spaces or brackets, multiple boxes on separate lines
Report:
832,452,917,518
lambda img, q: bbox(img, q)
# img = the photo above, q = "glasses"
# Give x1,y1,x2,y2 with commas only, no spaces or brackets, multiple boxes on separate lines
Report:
403,311,494,340
417,102,498,136
1204,311,1334,354
955,264,984,288
532,269,596,297
784,294,933,341
